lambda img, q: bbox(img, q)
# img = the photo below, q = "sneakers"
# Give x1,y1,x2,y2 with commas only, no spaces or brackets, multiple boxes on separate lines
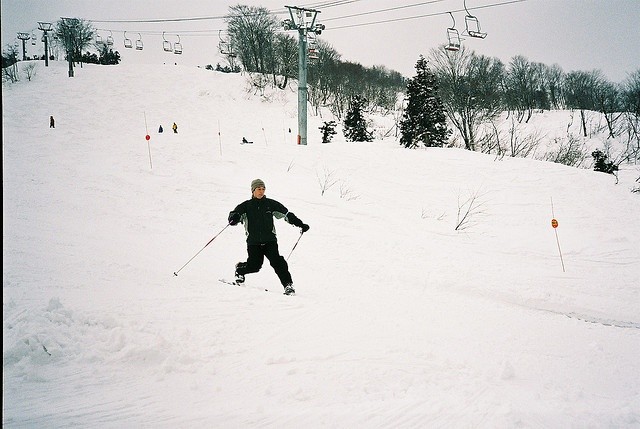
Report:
235,262,245,283
284,283,295,294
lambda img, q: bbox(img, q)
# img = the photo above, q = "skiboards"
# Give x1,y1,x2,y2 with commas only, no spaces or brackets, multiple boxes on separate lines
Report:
219,279,297,295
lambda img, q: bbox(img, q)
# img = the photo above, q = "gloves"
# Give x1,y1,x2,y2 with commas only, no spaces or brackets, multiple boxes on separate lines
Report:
300,223,309,233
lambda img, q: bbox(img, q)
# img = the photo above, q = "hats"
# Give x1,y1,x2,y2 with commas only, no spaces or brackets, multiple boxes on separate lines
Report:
251,179,265,193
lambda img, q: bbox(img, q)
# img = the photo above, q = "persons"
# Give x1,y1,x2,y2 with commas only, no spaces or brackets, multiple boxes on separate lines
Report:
159,126,163,133
289,128,291,133
173,122,178,134
227,178,310,296
241,137,248,144
49,116,54,128
